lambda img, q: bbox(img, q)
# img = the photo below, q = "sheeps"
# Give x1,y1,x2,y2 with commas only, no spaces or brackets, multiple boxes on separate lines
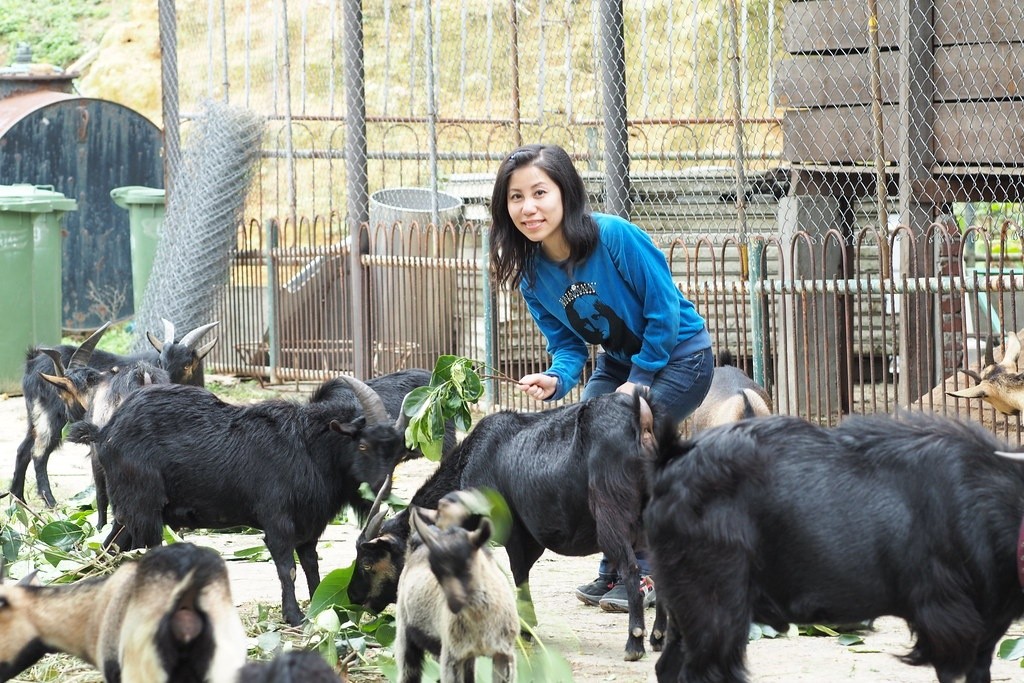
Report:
1,543,249,681
642,327,1024,683
60,368,459,629
346,349,774,683
10,317,221,534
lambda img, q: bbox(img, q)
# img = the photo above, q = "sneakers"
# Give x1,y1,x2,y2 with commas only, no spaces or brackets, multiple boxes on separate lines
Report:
599,574,656,613
575,577,617,605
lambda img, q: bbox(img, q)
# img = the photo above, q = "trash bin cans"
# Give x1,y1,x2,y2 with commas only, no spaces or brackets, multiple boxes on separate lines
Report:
110,184,169,318
0,181,79,398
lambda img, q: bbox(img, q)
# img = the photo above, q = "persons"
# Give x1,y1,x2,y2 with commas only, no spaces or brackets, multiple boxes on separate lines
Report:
490,143,715,610
